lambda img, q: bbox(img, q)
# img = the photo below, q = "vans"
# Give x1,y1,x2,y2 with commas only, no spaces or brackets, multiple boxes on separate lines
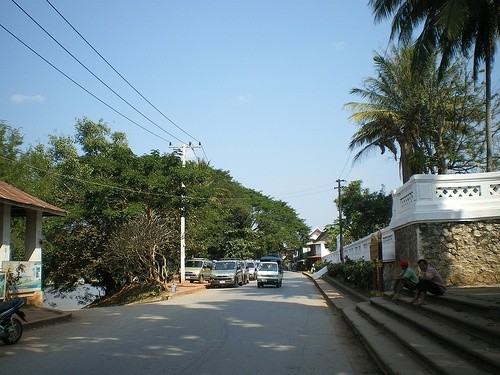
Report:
255,255,283,288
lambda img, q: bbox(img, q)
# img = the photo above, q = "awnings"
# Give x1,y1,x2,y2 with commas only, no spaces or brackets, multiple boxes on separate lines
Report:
307,256,321,258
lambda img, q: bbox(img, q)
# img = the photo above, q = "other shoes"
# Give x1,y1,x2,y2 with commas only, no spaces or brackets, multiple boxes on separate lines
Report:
388,292,401,298
406,299,417,303
413,300,427,305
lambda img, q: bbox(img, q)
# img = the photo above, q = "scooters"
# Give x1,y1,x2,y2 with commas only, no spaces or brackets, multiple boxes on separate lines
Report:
0,298,27,345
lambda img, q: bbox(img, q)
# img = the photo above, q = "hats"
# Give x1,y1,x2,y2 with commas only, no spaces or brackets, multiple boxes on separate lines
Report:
399,259,408,265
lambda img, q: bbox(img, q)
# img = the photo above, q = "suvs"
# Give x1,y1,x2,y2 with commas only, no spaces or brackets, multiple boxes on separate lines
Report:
178,257,213,284
208,258,260,288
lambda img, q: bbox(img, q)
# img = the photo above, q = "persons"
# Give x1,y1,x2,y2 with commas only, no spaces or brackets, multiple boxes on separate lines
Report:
389,259,419,299
406,259,446,305
